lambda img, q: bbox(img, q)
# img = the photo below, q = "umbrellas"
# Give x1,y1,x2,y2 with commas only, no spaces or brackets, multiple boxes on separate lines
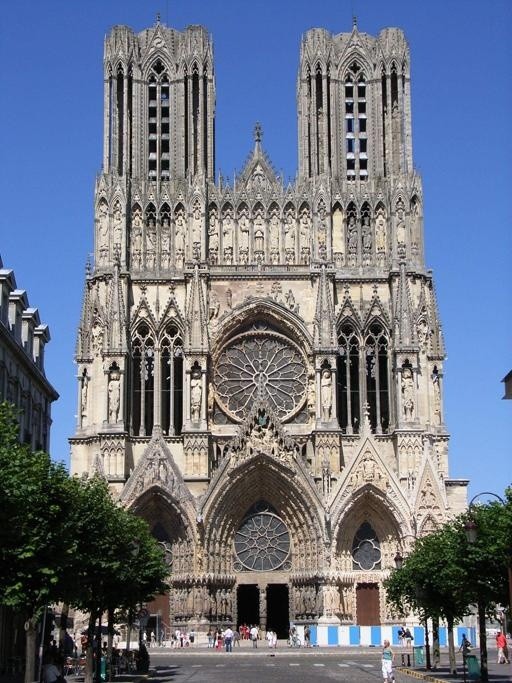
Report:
82,625,120,636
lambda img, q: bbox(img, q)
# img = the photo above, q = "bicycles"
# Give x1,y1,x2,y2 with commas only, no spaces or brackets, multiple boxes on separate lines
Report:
287,634,301,648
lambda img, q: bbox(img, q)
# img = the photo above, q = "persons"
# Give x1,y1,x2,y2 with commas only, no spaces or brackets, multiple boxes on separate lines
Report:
459,633,473,665
398,626,413,650
495,629,509,664
41,633,117,683
141,630,199,648
303,625,310,647
288,627,297,647
381,639,396,683
206,622,279,651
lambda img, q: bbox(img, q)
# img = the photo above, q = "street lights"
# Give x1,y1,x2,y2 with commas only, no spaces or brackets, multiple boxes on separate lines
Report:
394,534,430,669
464,492,507,544
